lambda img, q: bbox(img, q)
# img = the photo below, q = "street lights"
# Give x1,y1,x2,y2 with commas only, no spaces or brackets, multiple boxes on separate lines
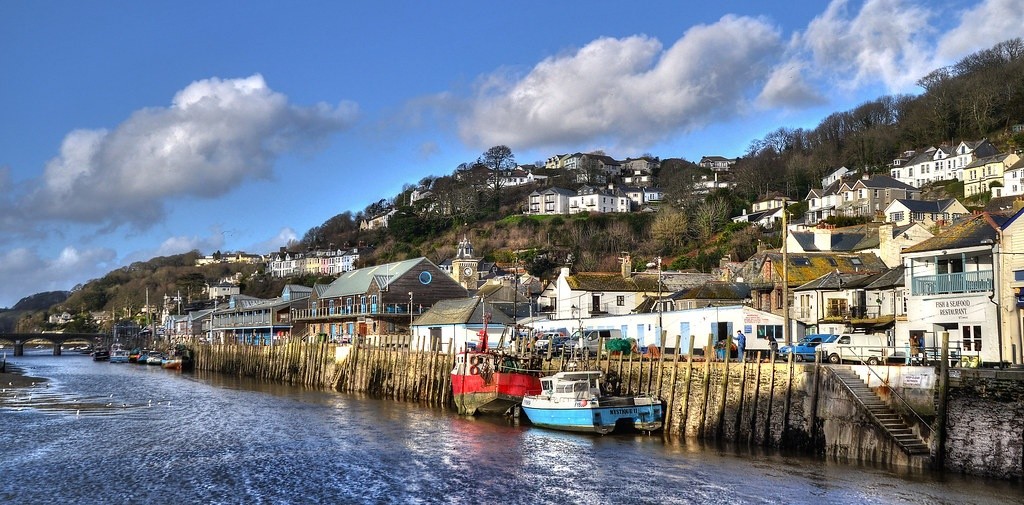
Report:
646,256,663,331
408,291,414,350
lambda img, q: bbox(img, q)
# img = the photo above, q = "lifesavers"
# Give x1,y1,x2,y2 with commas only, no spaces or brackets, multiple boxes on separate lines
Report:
470,356,479,365
470,365,478,376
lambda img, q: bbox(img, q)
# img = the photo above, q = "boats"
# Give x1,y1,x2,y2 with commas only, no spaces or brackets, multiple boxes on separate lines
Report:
449,292,542,421
520,326,663,437
61,340,184,371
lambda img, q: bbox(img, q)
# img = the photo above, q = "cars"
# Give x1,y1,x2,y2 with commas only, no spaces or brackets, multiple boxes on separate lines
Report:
541,335,570,358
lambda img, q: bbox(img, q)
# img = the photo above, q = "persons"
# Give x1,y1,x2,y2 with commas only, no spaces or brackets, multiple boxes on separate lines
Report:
907,334,930,366
732,330,746,362
765,335,778,363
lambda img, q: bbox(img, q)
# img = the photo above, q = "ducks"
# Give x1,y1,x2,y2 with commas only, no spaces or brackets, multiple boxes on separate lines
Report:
2,382,172,415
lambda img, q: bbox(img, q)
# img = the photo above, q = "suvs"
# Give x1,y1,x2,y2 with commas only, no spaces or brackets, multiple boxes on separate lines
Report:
778,333,840,363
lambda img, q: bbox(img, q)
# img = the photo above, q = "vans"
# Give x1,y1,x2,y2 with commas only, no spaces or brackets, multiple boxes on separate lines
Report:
562,327,622,359
512,330,566,356
814,332,890,366
